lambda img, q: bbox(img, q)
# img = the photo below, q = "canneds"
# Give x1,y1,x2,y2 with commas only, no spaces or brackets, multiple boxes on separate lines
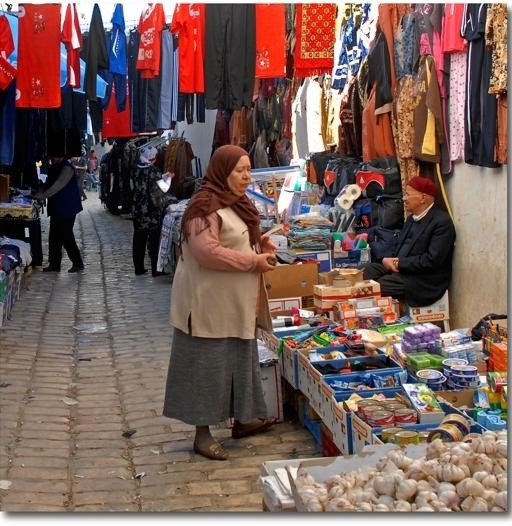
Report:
358,399,471,449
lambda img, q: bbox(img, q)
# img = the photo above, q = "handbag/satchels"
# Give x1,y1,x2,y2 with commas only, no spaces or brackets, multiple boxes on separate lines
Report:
306,152,401,197
151,186,174,207
355,193,404,229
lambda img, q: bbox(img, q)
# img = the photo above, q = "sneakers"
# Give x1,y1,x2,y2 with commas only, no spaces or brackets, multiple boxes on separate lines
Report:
232,417,279,438
44,264,84,273
193,439,230,459
135,269,170,277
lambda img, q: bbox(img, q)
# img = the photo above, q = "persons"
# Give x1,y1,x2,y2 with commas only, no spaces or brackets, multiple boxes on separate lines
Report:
31,157,87,273
130,145,177,276
356,175,455,307
70,143,101,201
162,144,280,462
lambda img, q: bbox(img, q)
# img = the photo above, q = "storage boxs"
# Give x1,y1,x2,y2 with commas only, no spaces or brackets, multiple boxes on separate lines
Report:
226,240,508,457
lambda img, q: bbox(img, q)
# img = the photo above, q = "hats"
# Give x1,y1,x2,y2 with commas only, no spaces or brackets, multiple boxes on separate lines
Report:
407,176,438,196
140,145,157,160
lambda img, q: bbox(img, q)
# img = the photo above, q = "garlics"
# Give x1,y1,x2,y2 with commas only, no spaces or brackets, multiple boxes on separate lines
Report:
294,429,508,512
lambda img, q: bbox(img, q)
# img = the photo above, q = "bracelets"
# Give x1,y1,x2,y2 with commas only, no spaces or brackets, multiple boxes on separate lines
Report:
392,260,398,268
